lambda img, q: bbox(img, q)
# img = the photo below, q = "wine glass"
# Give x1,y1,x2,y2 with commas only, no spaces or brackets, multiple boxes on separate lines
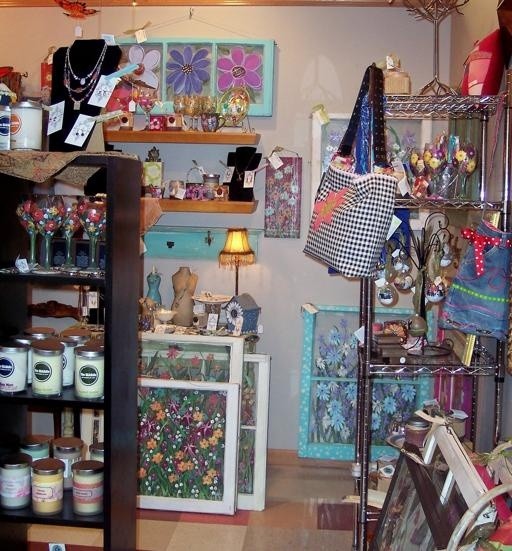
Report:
409,141,477,202
174,96,212,132
15,193,108,272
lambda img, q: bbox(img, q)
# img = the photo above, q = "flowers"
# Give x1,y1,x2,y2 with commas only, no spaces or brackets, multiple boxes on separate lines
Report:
308,315,420,447
265,164,300,238
163,41,213,97
323,122,480,184
119,42,160,92
14,193,107,240
215,44,264,95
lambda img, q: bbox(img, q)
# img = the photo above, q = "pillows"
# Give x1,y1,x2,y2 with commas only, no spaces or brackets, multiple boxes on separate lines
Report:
459,26,504,112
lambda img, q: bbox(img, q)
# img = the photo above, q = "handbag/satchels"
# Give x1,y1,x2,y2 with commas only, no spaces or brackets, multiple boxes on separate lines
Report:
301,64,411,281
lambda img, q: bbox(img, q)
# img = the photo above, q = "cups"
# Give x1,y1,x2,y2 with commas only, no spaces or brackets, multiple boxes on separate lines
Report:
164,116,182,131
201,113,226,132
168,181,185,201
149,116,164,131
185,183,202,199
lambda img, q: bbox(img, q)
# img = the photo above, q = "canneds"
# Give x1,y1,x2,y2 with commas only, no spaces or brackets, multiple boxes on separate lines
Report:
0,328,105,400
202,174,219,199
0,101,43,150
0,436,104,518
170,179,184,198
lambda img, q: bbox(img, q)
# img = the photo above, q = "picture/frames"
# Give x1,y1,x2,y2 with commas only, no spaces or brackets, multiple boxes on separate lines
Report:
392,417,498,551
69,35,277,119
309,107,433,235
134,328,274,519
294,302,436,469
367,441,457,551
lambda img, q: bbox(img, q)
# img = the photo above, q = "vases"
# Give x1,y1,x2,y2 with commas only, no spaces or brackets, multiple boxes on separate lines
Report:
27,227,103,273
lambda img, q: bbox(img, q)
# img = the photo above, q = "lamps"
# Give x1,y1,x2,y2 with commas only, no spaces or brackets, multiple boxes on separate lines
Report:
218,227,256,298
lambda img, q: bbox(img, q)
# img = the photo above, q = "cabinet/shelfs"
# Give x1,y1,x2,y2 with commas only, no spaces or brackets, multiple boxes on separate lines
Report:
0,146,144,551
351,68,511,551
100,126,261,215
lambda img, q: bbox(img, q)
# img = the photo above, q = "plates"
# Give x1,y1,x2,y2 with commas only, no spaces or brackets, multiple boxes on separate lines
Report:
386,434,424,454
217,88,251,127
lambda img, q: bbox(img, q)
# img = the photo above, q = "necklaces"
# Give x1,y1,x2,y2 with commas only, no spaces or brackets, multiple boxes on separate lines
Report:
64,41,108,109
